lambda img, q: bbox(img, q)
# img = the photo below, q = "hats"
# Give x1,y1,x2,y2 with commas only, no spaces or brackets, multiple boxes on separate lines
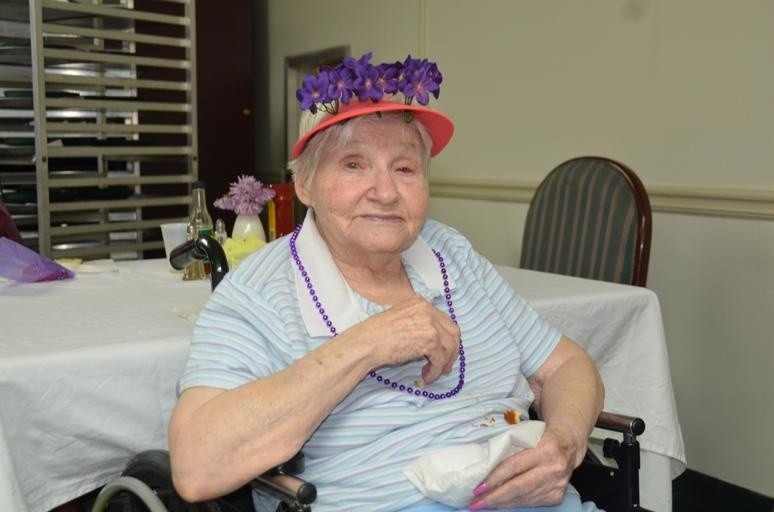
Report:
290,97,455,158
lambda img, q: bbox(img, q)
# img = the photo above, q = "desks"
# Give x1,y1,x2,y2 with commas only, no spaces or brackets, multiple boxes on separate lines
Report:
0,255,655,511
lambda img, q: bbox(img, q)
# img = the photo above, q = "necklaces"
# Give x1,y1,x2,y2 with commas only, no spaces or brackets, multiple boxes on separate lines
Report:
289,221,466,400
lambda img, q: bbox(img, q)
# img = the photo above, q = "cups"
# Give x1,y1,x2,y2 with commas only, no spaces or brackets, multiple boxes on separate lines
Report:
159,222,188,273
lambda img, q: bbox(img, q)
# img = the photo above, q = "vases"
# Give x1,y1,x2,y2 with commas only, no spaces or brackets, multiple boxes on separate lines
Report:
231,214,266,244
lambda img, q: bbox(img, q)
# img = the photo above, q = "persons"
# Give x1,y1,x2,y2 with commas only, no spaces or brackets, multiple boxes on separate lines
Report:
161,51,618,511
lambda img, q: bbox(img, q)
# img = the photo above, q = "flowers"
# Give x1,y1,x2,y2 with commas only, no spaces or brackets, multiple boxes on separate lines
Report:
294,49,442,124
212,173,276,216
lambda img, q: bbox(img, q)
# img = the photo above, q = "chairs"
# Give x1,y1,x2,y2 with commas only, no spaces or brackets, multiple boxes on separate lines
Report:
516,155,653,286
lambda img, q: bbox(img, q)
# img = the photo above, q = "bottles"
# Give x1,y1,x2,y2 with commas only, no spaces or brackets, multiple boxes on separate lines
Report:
183,180,228,282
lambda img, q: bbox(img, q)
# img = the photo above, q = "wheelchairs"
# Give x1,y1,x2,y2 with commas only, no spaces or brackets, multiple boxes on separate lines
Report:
90,240,655,511
